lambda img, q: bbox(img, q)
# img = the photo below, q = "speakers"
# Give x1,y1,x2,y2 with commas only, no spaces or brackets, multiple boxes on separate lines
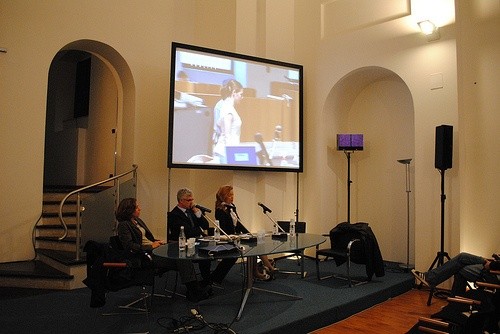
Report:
434,125,454,170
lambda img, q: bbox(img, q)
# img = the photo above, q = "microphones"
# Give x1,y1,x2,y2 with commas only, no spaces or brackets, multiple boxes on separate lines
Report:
258,202,272,213
255,132,272,166
192,203,211,213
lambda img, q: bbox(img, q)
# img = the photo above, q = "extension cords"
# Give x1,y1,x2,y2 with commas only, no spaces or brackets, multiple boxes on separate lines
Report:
190,309,203,320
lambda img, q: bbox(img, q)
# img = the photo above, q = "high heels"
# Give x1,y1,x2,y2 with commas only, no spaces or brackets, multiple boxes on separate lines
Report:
255,273,270,280
263,265,279,274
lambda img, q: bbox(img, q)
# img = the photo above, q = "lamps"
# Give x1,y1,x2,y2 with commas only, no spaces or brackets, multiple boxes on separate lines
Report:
335,134,364,223
397,158,414,269
417,20,441,42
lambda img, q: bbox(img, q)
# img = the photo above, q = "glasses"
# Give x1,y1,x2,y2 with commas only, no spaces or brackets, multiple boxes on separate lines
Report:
182,199,194,202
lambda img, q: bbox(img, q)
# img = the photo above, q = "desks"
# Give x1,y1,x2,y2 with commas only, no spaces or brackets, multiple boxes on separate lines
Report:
234,141,299,167
152,233,328,321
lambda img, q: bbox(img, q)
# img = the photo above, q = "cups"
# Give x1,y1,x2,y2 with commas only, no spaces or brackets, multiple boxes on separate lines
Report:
187,237,196,248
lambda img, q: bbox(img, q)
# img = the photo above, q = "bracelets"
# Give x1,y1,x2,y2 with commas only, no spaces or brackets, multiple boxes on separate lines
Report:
194,209,199,212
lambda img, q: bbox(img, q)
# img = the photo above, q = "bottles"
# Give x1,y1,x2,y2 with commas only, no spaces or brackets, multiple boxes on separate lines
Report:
214,220,220,241
289,218,295,237
273,218,278,234
179,226,185,251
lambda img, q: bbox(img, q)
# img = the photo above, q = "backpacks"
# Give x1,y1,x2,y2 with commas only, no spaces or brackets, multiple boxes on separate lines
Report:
448,289,497,334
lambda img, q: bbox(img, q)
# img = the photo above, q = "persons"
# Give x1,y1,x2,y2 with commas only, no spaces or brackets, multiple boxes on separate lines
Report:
211,79,245,163
163,188,239,296
411,252,500,297
214,185,280,279
114,196,205,301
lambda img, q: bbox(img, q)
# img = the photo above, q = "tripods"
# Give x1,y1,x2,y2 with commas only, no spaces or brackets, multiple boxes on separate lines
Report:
417,170,472,307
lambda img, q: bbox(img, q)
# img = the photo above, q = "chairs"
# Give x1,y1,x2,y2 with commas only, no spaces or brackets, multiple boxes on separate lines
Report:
315,223,386,288
83,240,150,315
276,221,307,278
419,270,500,334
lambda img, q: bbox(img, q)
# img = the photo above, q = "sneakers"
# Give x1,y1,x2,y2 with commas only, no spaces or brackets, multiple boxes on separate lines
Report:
410,269,431,287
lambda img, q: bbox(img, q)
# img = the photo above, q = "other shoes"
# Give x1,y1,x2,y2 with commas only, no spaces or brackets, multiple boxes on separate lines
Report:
184,287,213,302
206,279,225,289
